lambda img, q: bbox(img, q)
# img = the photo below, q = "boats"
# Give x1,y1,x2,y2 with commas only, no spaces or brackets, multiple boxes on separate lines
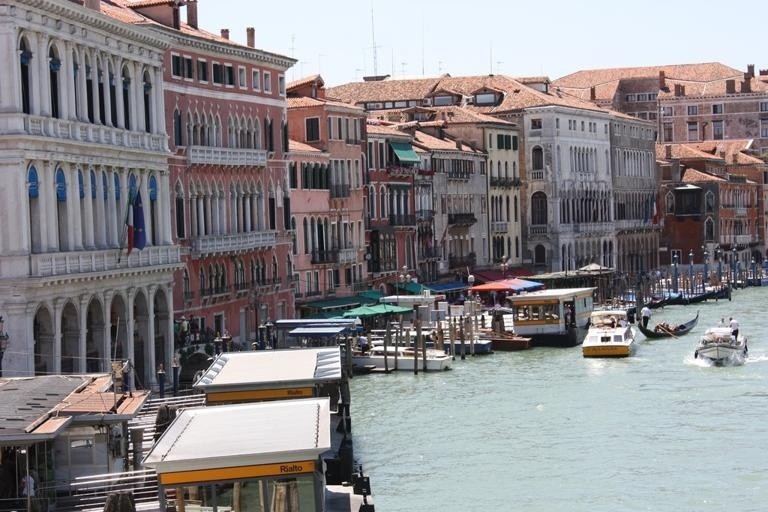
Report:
639,309,701,339
615,256,748,309
694,325,749,366
581,318,636,356
353,346,454,372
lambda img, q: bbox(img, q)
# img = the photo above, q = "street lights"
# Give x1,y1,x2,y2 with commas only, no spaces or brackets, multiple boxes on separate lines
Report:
498,256,509,281
0,315,8,377
399,265,411,297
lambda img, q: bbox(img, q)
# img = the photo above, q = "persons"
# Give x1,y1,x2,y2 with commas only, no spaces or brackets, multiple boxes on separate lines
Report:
618,317,626,328
19,468,36,512
188,314,196,344
728,317,739,345
607,314,617,327
716,318,727,327
640,304,651,329
657,323,674,332
653,322,665,333
353,334,370,353
175,315,187,348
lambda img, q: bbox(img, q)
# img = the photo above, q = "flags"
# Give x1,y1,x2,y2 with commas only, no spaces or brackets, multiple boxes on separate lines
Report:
134,190,147,251
124,188,135,255
